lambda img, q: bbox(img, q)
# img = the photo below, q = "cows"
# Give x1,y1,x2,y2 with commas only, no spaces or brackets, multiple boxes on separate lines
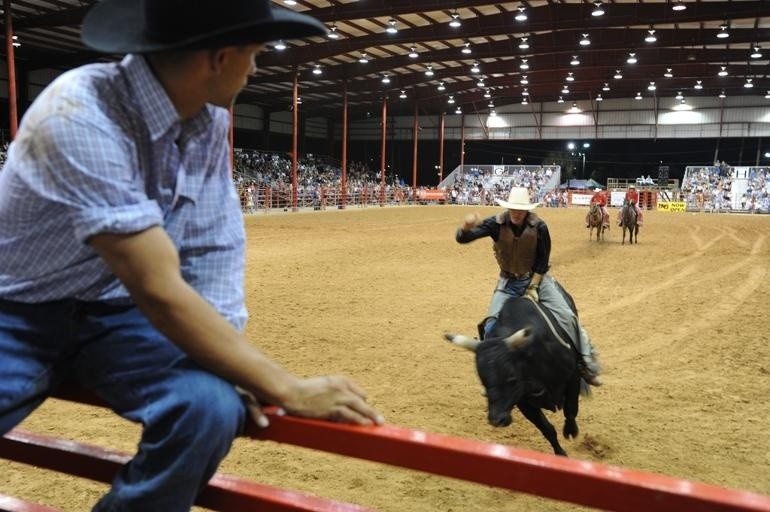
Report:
444,281,582,457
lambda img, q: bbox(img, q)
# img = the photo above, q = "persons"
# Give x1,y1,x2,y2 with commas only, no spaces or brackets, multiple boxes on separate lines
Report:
586,187,609,229
454,187,605,389
231,142,769,216
617,185,643,226
0,2,381,512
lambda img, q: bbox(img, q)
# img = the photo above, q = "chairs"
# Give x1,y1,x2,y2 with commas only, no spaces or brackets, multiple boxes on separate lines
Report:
682,171,770,212
444,166,554,205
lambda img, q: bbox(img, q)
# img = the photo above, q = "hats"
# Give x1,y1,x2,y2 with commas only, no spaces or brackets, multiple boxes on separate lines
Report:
592,187,602,193
73,1,331,59
493,187,541,210
629,184,636,189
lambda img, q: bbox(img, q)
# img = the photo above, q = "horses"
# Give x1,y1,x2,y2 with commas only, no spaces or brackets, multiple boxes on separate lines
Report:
589,199,605,242
621,197,639,245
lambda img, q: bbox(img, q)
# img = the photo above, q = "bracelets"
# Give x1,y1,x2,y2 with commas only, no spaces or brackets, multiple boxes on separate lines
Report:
527,283,539,290
